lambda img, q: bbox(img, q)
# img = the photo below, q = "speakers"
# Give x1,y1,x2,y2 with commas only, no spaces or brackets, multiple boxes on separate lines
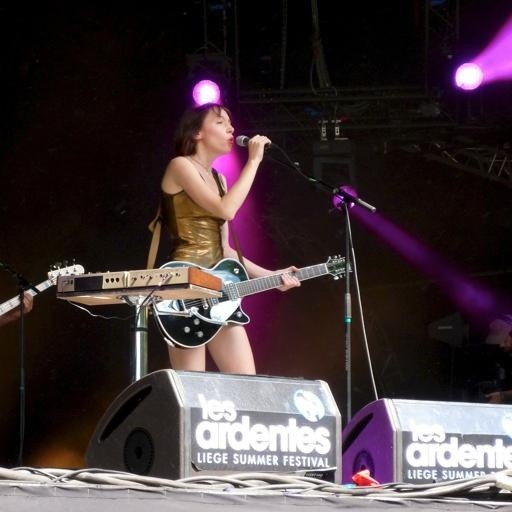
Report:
341,397,512,492
85,368,343,484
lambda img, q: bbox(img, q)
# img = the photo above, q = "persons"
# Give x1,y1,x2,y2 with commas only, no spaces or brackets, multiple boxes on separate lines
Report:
158,101,301,378
479,312,512,404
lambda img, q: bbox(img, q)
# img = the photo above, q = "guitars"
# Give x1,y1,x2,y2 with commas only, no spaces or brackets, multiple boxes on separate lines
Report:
150,254,353,348
0,259,85,315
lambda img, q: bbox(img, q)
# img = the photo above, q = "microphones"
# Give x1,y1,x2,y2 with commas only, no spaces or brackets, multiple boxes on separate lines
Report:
236,135,271,150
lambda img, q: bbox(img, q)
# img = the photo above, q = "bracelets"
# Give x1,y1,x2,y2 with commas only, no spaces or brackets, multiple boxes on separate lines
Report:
500,391,506,402
